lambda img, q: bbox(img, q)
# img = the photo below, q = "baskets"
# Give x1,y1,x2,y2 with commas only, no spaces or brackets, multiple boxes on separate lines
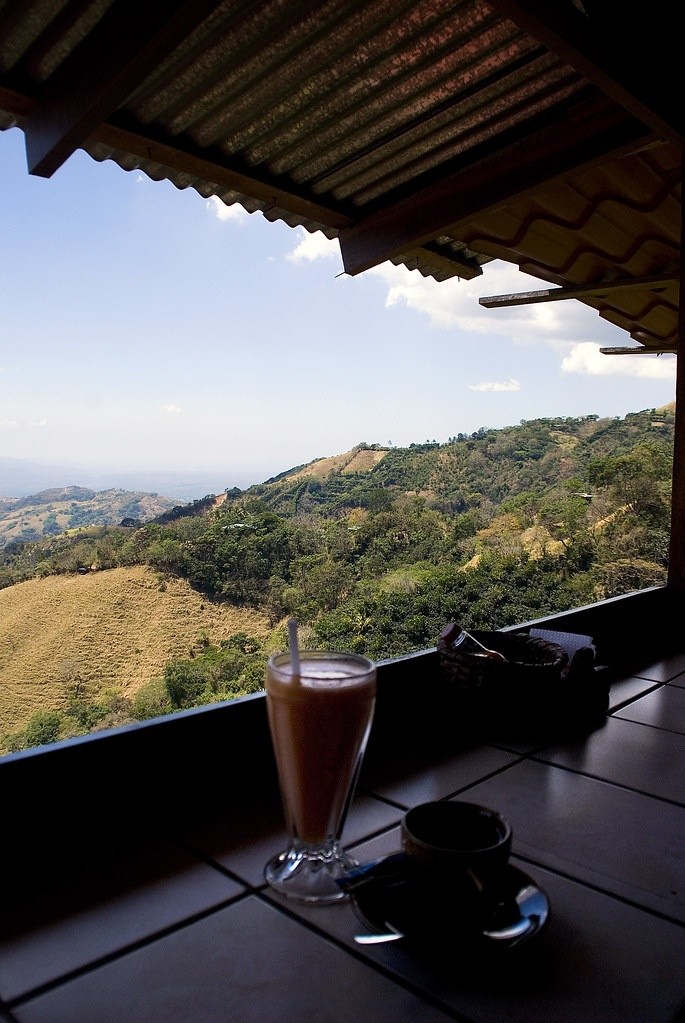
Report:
437,628,569,698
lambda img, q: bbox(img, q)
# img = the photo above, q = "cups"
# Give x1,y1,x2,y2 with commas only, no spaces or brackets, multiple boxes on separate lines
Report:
400,800,514,921
262,645,385,906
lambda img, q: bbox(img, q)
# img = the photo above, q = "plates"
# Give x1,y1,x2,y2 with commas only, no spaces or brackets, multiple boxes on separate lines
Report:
348,848,551,951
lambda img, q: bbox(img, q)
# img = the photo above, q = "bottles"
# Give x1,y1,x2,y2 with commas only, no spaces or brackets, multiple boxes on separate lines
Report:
441,623,507,662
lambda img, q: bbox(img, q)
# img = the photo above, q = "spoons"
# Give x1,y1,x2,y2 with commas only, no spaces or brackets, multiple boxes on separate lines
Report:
353,913,535,947
